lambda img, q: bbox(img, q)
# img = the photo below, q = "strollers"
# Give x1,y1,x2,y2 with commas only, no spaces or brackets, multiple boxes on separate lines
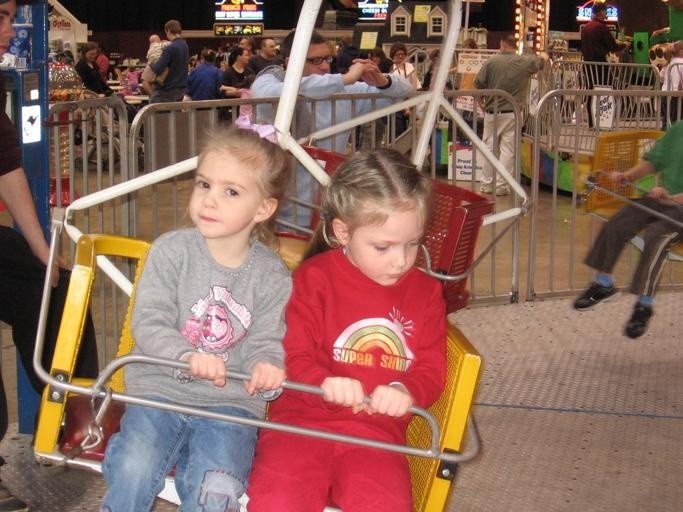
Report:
72,88,145,172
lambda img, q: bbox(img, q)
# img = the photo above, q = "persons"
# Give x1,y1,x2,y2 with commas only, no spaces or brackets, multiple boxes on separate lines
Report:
573,120,683,338
246,148,447,512
581,0,631,128
0,0,99,512
100,128,293,512
659,40,683,126
473,31,549,196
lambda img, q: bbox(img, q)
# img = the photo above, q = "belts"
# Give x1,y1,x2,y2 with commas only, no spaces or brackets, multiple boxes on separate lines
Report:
486,109,513,114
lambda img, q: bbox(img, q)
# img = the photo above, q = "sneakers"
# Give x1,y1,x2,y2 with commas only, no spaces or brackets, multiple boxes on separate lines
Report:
480,184,492,193
574,281,619,307
623,304,653,338
0,481,28,512
496,188,513,195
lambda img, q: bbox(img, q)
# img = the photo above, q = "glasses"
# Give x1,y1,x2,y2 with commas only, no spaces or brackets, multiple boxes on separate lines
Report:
306,53,335,64
